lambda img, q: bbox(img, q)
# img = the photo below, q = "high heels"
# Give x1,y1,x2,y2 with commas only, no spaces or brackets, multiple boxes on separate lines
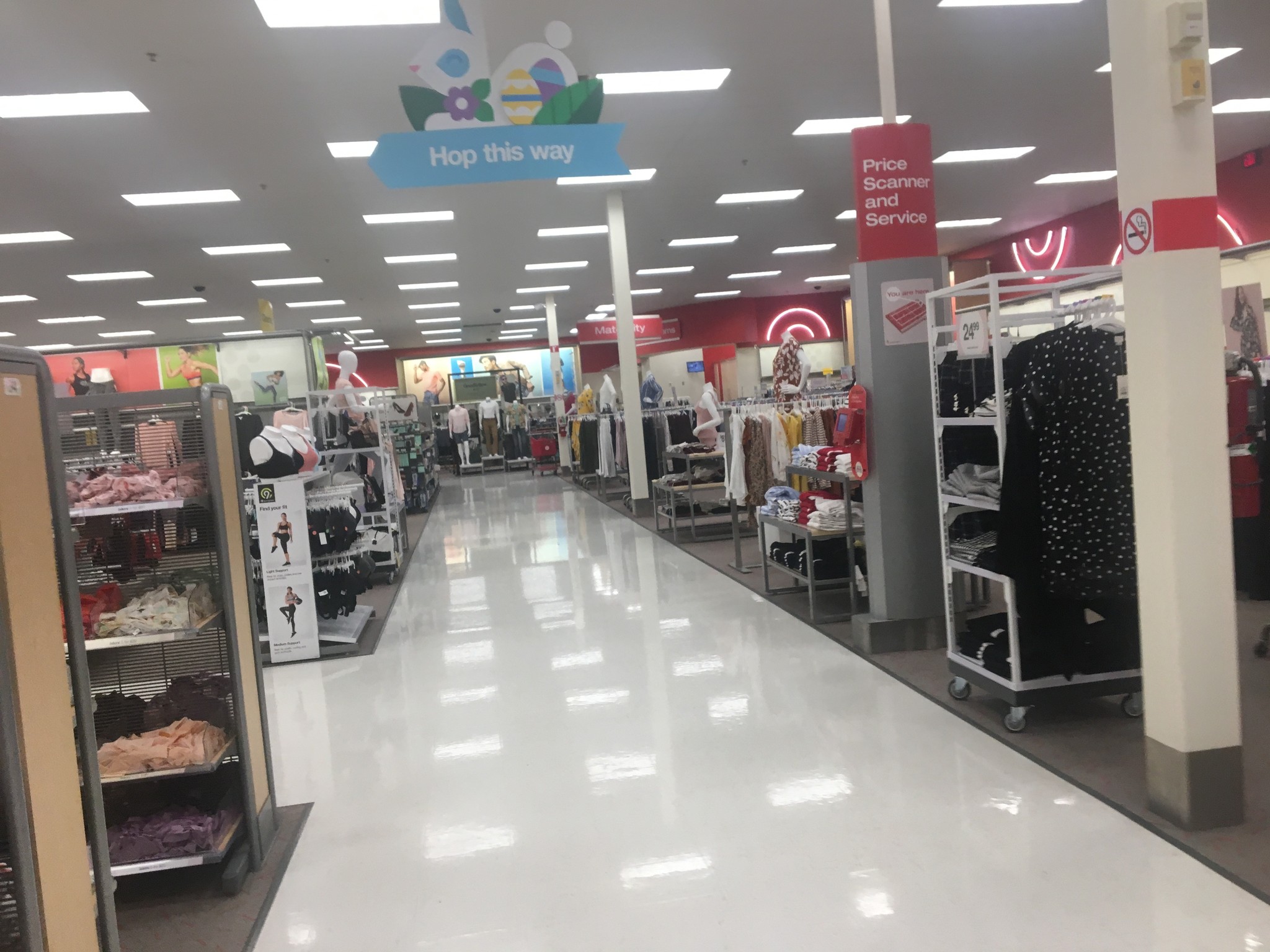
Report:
403,402,414,416
393,401,405,413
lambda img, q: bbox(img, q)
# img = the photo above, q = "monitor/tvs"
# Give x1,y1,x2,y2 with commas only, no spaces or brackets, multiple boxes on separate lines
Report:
687,361,704,373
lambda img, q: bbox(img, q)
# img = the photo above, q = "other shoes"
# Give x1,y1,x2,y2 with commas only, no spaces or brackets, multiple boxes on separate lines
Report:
282,562,291,566
286,616,292,625
271,546,277,553
290,632,296,638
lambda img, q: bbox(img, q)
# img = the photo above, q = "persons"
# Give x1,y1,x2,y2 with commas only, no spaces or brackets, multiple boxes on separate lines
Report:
279,425,318,477
564,390,576,418
271,513,293,566
772,330,811,416
165,344,218,387
249,425,304,485
599,373,616,413
65,356,91,396
448,405,471,466
478,396,500,457
506,400,528,460
301,426,322,474
326,350,388,509
279,587,302,638
499,374,517,408
413,360,446,405
641,370,664,409
254,371,283,404
90,369,122,455
478,355,533,399
693,381,724,447
578,383,594,414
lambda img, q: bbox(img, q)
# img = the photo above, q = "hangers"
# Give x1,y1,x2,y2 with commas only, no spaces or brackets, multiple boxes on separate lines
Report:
242,485,370,580
148,402,303,423
1064,297,1125,334
569,385,850,422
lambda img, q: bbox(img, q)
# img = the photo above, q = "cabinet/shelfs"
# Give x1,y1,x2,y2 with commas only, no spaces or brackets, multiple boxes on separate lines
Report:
926,263,1143,732
651,451,758,542
306,386,403,584
1,349,277,952
759,465,870,624
368,393,440,515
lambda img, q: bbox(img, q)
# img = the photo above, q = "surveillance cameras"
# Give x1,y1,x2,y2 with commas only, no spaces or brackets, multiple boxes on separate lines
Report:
486,338,492,341
493,308,501,313
814,285,821,290
193,286,205,292
